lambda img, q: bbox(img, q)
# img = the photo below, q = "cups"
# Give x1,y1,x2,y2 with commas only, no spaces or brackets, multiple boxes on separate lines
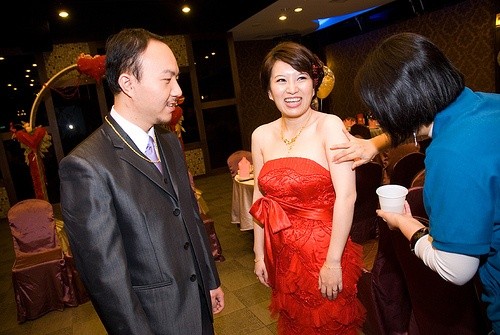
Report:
376,185,408,222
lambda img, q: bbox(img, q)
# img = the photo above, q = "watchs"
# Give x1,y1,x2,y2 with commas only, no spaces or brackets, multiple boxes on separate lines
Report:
253,258,265,262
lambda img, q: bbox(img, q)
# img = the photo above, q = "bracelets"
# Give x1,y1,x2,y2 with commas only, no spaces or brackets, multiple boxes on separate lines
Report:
408,226,430,255
322,262,343,270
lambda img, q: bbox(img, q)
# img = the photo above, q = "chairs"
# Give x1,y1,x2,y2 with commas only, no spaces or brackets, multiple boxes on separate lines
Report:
342,116,494,335
7,150,252,322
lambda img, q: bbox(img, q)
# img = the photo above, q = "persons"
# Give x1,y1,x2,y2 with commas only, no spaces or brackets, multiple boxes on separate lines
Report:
251,41,364,335
329,31,500,335
57,27,226,335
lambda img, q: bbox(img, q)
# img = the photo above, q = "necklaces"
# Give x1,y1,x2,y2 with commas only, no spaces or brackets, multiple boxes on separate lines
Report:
279,107,312,150
104,116,162,163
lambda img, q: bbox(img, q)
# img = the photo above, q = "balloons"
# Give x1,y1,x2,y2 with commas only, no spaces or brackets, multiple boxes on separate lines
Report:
315,65,335,97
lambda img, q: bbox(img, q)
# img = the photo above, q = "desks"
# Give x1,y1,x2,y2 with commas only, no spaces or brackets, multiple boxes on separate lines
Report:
230,174,254,231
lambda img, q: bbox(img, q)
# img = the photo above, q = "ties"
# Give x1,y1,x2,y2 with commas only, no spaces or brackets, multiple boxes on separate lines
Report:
145,135,164,175
